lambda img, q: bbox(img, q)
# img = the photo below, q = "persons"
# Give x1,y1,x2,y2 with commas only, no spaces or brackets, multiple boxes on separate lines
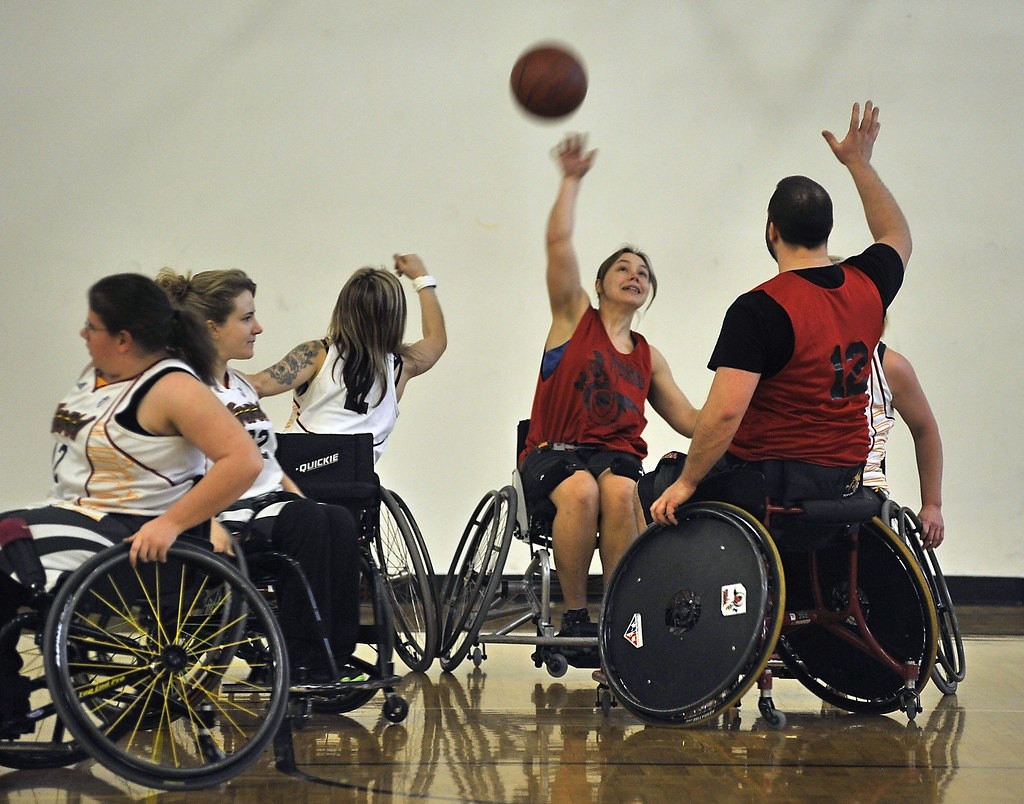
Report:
637,101,913,528
232,253,449,474
153,266,365,686
516,130,703,657
0,273,261,737
827,254,945,549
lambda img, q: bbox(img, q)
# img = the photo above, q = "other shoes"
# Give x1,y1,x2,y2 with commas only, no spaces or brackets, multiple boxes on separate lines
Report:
291,657,364,684
0,698,35,739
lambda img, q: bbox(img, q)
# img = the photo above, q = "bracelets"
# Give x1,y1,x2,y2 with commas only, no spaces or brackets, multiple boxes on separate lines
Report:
412,276,436,292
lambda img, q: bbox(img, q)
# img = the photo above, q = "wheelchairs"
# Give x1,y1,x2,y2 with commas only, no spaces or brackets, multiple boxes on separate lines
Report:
0,475,289,789
776,498,966,696
439,419,597,678
597,457,938,732
276,433,440,671
72,493,410,727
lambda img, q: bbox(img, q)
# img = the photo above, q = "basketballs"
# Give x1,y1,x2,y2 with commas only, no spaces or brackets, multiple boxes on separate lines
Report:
509,45,588,119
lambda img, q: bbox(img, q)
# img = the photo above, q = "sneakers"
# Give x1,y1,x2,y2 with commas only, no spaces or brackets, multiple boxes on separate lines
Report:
558,608,597,657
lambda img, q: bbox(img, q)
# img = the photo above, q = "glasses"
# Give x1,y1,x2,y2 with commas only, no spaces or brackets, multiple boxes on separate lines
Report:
84,321,108,333
